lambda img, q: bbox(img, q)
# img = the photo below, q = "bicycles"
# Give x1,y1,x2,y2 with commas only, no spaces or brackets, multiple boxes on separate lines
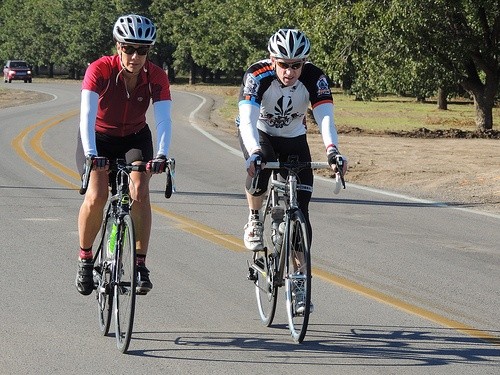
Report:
79,154,180,355
245,151,348,344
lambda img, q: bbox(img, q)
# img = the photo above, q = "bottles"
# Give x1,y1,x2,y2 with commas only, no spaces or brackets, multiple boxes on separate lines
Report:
110,218,128,257
275,221,286,254
105,214,117,262
272,203,285,246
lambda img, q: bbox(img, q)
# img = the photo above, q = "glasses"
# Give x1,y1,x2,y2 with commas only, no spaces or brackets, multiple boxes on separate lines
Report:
121,43,149,55
275,60,305,70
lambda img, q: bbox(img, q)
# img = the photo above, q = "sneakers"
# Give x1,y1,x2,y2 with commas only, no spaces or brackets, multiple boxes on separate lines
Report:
243,219,264,250
136,262,153,292
293,291,314,314
75,256,93,296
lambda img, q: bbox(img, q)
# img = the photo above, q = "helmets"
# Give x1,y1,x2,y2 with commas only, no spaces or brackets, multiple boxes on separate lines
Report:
113,15,156,45
268,29,311,60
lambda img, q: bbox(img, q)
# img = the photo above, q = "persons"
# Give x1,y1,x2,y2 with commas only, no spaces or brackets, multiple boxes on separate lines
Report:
75,15,172,296
235,28,349,314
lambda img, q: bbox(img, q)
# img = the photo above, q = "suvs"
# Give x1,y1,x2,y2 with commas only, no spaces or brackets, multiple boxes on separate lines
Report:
3,60,33,83
